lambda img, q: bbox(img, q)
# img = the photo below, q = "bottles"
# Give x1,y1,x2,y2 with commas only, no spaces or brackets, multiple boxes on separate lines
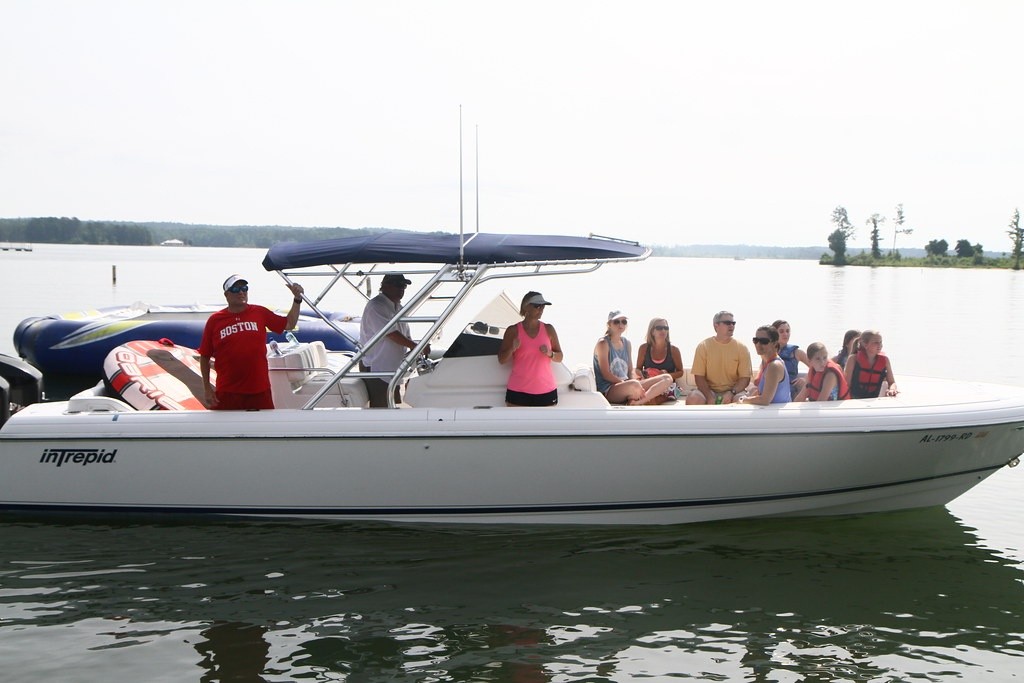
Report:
284,330,298,345
269,337,282,355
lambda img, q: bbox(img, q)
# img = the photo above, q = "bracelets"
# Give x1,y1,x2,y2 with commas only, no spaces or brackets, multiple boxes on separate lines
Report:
729,388,736,395
550,351,554,358
294,298,302,304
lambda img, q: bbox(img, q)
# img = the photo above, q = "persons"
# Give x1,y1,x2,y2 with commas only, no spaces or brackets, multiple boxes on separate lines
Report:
742,326,791,406
793,342,851,402
199,275,304,411
831,330,898,399
686,311,753,405
635,317,684,405
754,321,810,400
593,310,673,406
498,292,563,408
359,274,430,409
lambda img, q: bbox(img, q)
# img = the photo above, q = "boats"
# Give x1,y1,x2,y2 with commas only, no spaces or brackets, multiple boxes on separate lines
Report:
0,105,1024,531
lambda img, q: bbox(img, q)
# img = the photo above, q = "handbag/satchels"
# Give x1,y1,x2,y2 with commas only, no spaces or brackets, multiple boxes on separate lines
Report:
668,382,682,400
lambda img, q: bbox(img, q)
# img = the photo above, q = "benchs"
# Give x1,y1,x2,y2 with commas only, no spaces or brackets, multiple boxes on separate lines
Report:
286,340,369,406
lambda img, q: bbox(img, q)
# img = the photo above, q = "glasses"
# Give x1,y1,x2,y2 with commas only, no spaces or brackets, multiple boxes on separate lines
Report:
229,286,248,293
752,337,773,345
391,282,407,289
653,325,669,331
718,321,736,326
530,304,545,309
610,320,628,325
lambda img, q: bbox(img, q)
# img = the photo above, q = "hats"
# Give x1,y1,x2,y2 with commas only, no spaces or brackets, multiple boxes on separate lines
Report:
608,310,630,321
224,276,249,293
522,291,552,304
382,274,412,285
